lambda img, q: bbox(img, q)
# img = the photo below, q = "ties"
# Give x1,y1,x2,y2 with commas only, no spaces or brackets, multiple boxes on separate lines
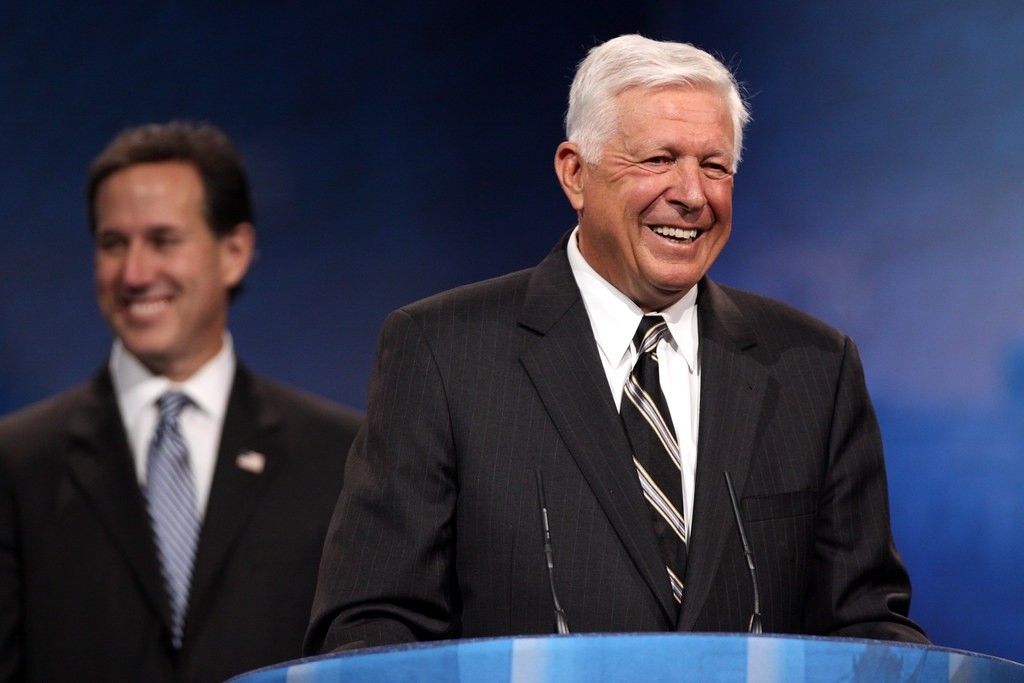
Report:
143,390,200,649
621,315,688,607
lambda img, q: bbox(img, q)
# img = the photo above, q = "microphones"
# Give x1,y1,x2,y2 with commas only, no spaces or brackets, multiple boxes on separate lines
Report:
724,471,763,635
535,468,570,635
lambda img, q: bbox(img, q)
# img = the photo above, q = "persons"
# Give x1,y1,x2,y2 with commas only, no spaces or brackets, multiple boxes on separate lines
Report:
0,119,369,682
298,34,927,657
